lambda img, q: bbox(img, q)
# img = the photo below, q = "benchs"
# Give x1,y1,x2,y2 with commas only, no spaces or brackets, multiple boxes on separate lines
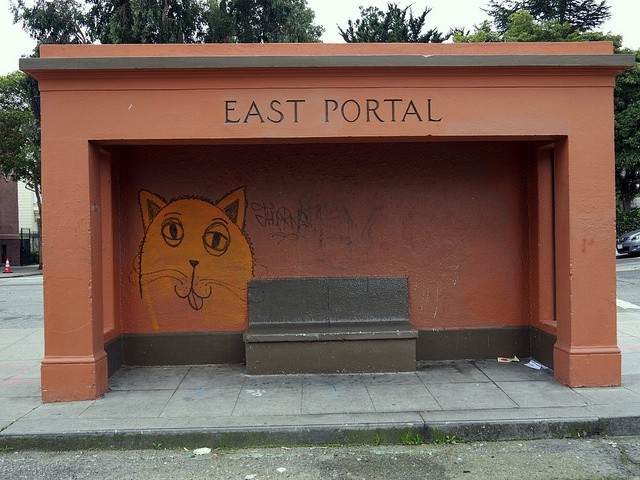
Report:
243,277,419,375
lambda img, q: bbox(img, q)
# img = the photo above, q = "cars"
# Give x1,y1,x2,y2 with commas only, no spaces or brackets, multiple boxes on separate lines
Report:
616,230,640,256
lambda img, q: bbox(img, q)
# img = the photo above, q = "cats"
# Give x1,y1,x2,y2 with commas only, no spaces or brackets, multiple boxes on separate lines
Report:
130,186,255,332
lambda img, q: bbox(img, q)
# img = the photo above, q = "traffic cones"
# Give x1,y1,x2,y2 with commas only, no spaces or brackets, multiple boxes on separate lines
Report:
2,256,12,273
